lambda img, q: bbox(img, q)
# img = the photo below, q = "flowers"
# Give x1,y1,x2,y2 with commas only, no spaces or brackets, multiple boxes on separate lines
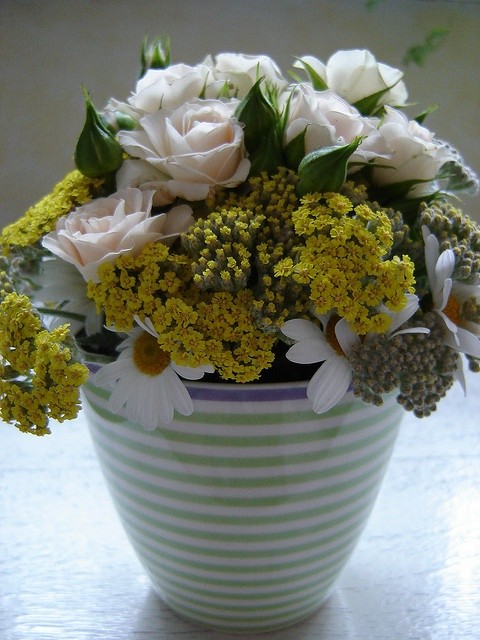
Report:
0,27,480,440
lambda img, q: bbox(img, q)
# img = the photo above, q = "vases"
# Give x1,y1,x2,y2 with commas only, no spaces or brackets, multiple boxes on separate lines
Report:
79,357,404,632
